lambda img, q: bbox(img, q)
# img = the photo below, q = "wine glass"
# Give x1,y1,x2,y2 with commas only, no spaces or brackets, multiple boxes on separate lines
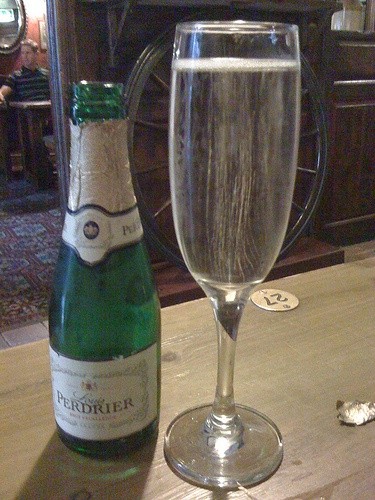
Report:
165,21,302,488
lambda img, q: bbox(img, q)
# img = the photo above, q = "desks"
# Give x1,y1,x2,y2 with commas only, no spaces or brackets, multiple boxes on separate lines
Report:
0,257,375,500
8,100,53,191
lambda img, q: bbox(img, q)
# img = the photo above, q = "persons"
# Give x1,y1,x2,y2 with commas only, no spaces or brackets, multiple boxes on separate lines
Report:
0,39,54,138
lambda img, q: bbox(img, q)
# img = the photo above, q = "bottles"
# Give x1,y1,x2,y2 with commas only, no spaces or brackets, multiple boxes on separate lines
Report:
49,81,162,460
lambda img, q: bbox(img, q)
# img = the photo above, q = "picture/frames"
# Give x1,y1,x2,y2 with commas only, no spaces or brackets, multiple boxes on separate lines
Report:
38,20,48,52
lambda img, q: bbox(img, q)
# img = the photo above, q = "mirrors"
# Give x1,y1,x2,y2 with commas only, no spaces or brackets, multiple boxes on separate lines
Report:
0,0,28,55
